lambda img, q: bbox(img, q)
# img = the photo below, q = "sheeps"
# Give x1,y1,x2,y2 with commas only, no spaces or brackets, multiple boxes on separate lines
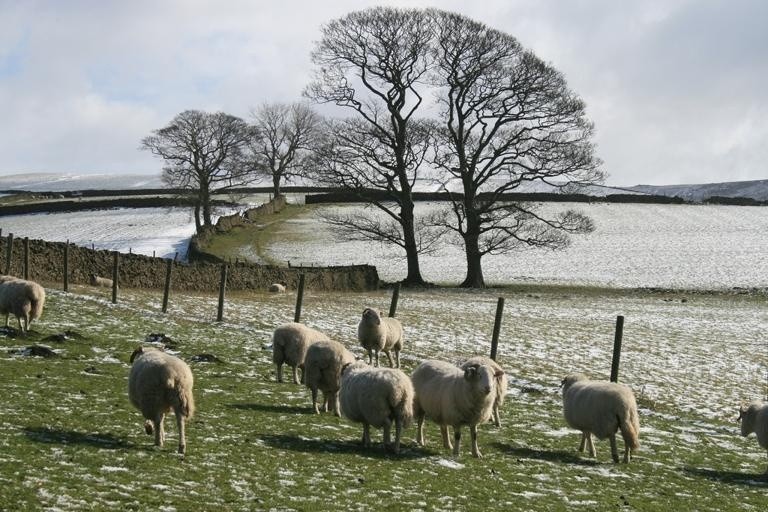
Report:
559,372,642,465
735,399,768,451
272,308,508,459
124,342,194,454
269,281,289,294
88,271,117,287
0,274,46,329
